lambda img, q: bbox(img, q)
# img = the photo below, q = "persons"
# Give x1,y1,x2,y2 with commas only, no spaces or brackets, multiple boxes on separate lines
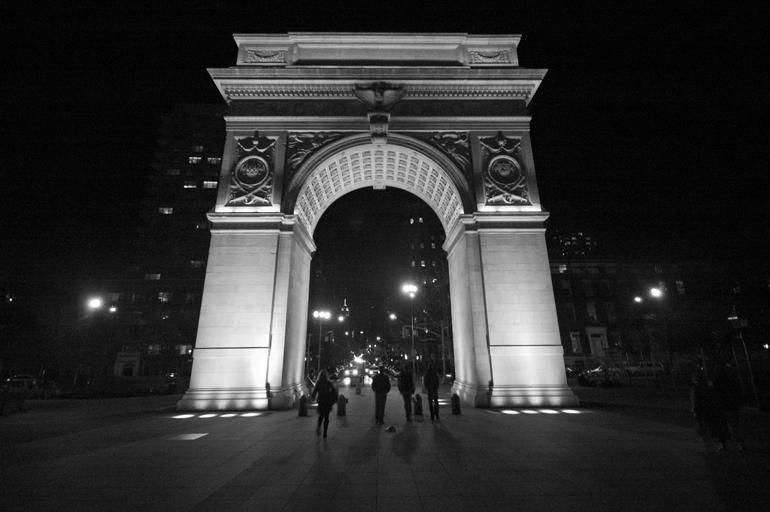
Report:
694,365,747,456
311,370,337,440
371,366,391,425
424,364,440,421
397,365,415,421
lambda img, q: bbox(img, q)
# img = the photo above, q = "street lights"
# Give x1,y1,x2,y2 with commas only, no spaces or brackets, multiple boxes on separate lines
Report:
310,307,329,372
396,275,420,377
647,285,678,392
402,320,445,375
72,294,104,384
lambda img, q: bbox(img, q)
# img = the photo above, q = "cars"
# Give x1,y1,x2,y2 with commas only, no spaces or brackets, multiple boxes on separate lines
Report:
608,360,664,377
5,373,47,386
